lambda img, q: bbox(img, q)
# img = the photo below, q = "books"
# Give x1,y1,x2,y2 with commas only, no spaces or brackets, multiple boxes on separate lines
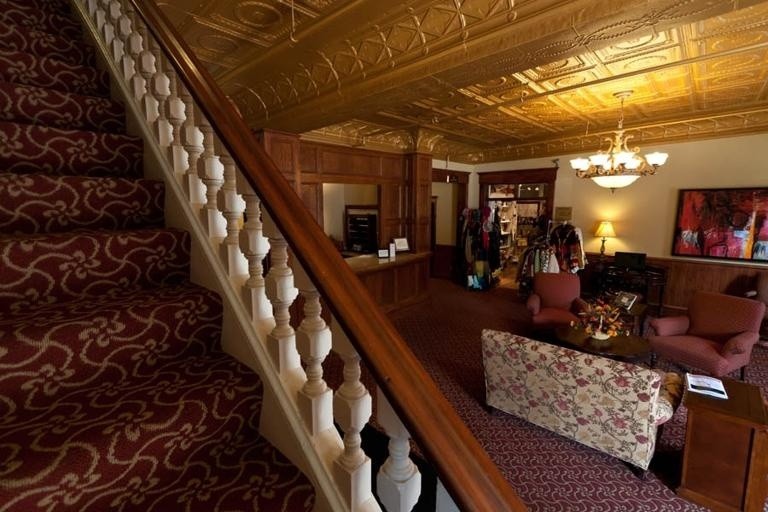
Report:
686,371,728,399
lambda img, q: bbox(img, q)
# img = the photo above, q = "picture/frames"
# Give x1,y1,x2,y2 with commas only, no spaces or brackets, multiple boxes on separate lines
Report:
669,188,768,264
614,291,637,310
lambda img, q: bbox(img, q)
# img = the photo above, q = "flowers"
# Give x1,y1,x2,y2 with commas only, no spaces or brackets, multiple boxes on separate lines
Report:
569,300,630,338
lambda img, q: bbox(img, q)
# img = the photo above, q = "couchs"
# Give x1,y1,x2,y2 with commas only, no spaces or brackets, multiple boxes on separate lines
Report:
478,315,684,480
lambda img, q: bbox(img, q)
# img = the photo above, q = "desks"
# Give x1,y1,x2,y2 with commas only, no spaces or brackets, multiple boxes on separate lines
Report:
596,298,647,337
584,255,667,317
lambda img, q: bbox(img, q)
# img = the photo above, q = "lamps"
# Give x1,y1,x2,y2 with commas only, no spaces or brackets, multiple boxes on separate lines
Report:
593,221,615,254
570,90,669,195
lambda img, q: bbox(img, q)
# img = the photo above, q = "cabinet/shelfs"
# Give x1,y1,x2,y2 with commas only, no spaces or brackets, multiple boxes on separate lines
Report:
488,184,518,266
514,201,542,253
676,381,768,512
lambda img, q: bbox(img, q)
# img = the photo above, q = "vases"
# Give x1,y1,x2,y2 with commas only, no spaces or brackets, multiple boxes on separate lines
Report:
592,330,610,340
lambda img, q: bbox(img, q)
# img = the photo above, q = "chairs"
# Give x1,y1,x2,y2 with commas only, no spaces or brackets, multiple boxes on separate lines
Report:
648,290,768,384
613,252,646,308
527,272,589,337
